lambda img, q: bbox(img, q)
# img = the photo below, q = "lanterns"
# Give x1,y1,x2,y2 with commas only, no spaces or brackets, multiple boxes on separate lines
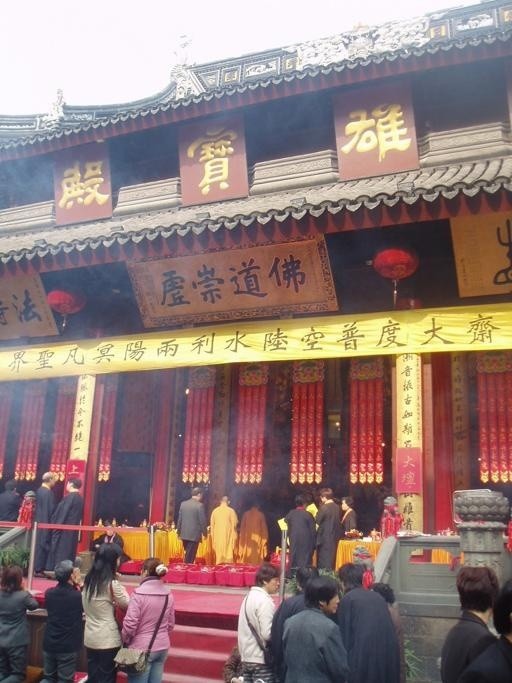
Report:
374,243,421,307
46,282,87,332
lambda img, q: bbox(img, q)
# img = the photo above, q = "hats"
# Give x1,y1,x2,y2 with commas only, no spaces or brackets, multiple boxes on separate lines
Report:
54,559,72,579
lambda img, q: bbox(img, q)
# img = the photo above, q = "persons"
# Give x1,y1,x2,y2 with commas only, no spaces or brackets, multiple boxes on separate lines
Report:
285,488,358,569
270,563,406,683
238,503,269,563
176,487,207,563
441,566,511,682
93,526,124,550
34,471,84,572
1,480,21,522
122,558,176,682
81,543,131,682
41,560,85,682
210,496,239,564
238,563,280,682
1,565,38,682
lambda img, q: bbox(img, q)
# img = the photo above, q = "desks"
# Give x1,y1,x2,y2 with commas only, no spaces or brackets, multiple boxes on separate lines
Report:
336,539,384,570
94,528,168,565
432,535,463,564
167,527,212,560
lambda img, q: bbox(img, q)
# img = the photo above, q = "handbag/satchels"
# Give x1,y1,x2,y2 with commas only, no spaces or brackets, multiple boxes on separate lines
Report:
264,647,274,668
114,648,145,673
